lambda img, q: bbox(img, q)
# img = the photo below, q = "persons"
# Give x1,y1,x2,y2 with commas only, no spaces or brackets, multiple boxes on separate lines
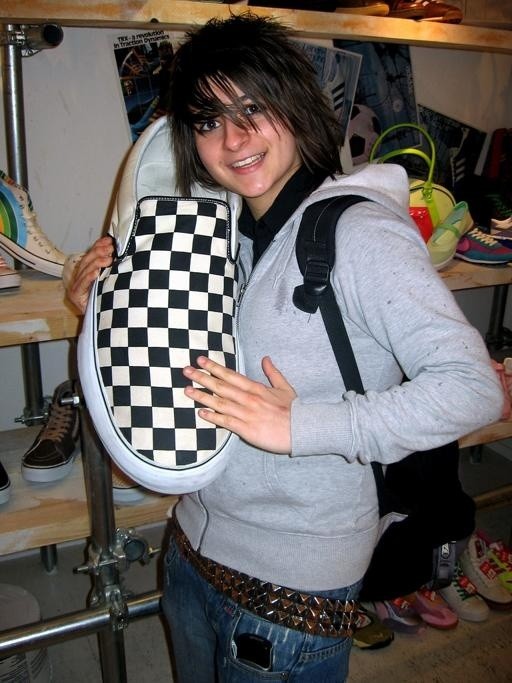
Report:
64,15,505,683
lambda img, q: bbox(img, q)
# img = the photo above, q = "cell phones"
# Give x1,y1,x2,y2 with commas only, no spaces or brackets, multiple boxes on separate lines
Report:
233,632,274,672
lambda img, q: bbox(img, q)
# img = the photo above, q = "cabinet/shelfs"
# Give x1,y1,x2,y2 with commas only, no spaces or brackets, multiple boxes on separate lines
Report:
0,2,511,678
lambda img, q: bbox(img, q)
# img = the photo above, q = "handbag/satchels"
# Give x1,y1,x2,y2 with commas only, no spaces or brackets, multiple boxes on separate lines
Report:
362,440,478,601
408,179,457,237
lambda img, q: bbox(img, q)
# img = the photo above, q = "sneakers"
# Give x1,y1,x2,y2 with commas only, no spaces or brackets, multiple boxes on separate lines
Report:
1,171,68,277
353,532,512,648
76,113,246,497
19,380,80,483
427,201,511,271
